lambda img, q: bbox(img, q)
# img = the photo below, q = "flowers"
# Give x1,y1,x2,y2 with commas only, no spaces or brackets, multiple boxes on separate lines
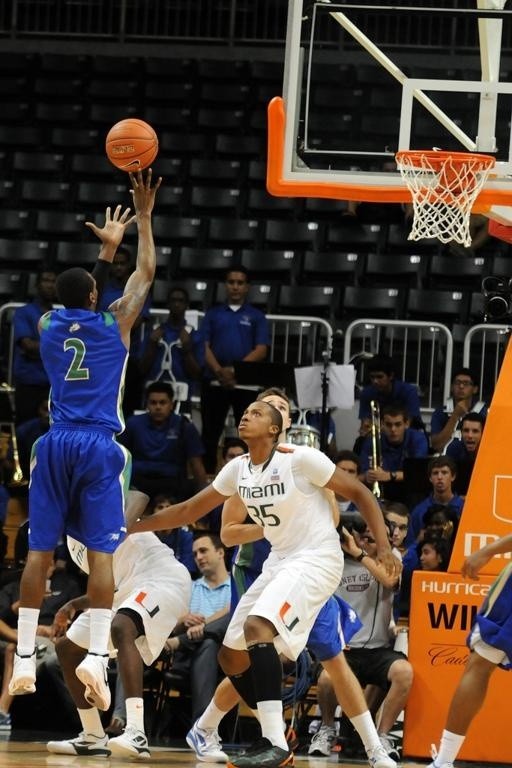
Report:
368,538,376,543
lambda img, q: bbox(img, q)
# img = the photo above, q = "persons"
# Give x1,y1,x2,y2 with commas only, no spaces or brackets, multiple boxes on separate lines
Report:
309,520,414,763
428,536,512,768
0,249,512,753
186,391,397,767
129,401,404,768
7,160,163,712
47,491,193,760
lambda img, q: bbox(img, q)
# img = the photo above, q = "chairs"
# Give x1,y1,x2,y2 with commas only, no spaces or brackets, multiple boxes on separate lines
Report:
46,717,151,761
0,711,13,731
228,721,299,768
185,719,229,764
307,725,338,757
8,653,36,696
75,652,112,712
368,736,400,768
0,54,512,404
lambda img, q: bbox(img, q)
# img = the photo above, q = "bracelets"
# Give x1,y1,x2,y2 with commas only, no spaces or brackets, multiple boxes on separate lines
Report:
358,549,368,561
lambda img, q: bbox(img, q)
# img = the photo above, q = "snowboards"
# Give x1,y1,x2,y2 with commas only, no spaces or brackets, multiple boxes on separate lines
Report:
106,119,158,172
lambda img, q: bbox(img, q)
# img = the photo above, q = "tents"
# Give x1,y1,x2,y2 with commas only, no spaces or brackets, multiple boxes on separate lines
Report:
384,518,394,546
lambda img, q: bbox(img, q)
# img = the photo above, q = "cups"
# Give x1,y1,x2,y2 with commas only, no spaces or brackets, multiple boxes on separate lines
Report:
453,379,473,386
